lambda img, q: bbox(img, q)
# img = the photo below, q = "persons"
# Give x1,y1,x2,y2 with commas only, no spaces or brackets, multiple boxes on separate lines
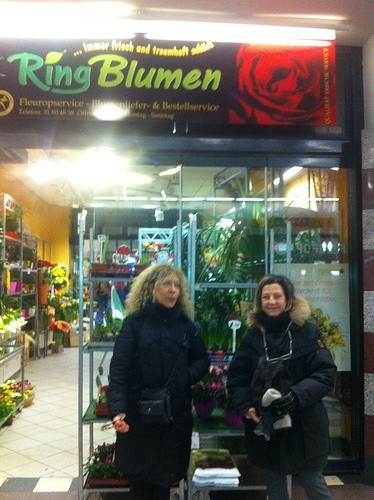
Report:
225,274,337,500
106,263,210,500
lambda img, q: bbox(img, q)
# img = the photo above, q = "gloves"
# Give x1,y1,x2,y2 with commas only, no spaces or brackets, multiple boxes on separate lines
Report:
254,391,300,441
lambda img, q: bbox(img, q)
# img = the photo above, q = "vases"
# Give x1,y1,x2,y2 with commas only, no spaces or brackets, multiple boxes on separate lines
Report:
54,330,63,345
90,263,146,277
86,480,129,489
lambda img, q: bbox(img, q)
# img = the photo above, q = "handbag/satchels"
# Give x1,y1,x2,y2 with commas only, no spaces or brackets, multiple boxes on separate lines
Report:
138,385,173,426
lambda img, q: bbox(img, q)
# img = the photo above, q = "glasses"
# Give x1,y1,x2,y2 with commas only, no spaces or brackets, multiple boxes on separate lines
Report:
264,340,293,365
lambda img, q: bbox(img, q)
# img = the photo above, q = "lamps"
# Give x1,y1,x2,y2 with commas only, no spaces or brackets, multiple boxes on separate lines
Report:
161,186,176,197
170,173,180,185
155,209,164,222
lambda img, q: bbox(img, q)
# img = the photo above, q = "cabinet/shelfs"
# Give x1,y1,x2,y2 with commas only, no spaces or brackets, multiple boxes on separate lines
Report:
0,190,38,428
76,167,341,500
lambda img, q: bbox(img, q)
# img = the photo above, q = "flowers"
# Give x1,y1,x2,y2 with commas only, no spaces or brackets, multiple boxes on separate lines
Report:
0,213,346,476
